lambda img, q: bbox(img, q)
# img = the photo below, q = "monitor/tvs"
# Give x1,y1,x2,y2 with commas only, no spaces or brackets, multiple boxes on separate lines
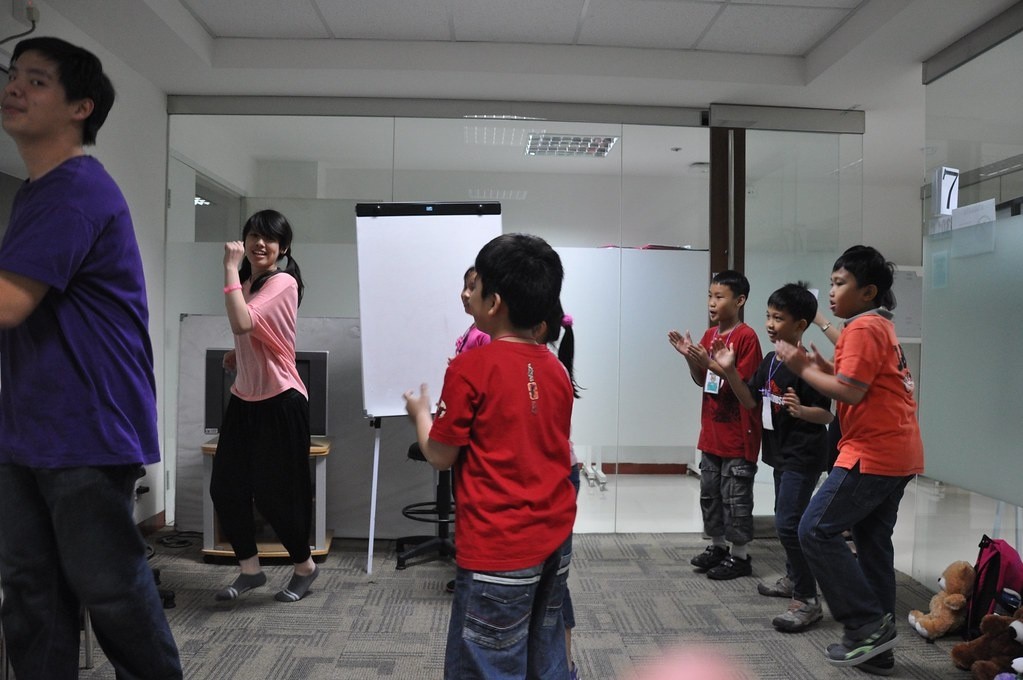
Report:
205,350,329,438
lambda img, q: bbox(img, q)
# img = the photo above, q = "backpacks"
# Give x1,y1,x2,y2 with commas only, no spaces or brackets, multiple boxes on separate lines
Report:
963,534,1023,641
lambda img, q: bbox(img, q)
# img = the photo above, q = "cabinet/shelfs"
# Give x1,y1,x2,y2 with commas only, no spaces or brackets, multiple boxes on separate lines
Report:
200,435,333,558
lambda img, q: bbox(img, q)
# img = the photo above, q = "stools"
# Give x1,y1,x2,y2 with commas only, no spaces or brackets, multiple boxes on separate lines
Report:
395,443,465,572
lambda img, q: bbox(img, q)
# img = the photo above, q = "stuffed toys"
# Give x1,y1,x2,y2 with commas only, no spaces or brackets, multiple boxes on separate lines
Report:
951,605,1023,680
909,561,974,640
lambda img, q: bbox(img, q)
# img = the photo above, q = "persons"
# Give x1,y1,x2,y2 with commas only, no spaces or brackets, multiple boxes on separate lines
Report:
210,210,319,603
1,38,187,679
711,280,835,634
667,271,762,580
403,233,586,680
772,242,923,673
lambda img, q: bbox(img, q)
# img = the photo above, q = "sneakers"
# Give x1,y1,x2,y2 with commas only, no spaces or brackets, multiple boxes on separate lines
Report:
690,545,731,568
757,576,802,600
825,643,895,676
772,598,824,633
707,554,752,581
827,612,901,666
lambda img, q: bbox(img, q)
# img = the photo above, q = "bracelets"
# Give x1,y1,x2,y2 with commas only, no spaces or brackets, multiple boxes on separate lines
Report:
223,284,243,294
822,322,831,331
